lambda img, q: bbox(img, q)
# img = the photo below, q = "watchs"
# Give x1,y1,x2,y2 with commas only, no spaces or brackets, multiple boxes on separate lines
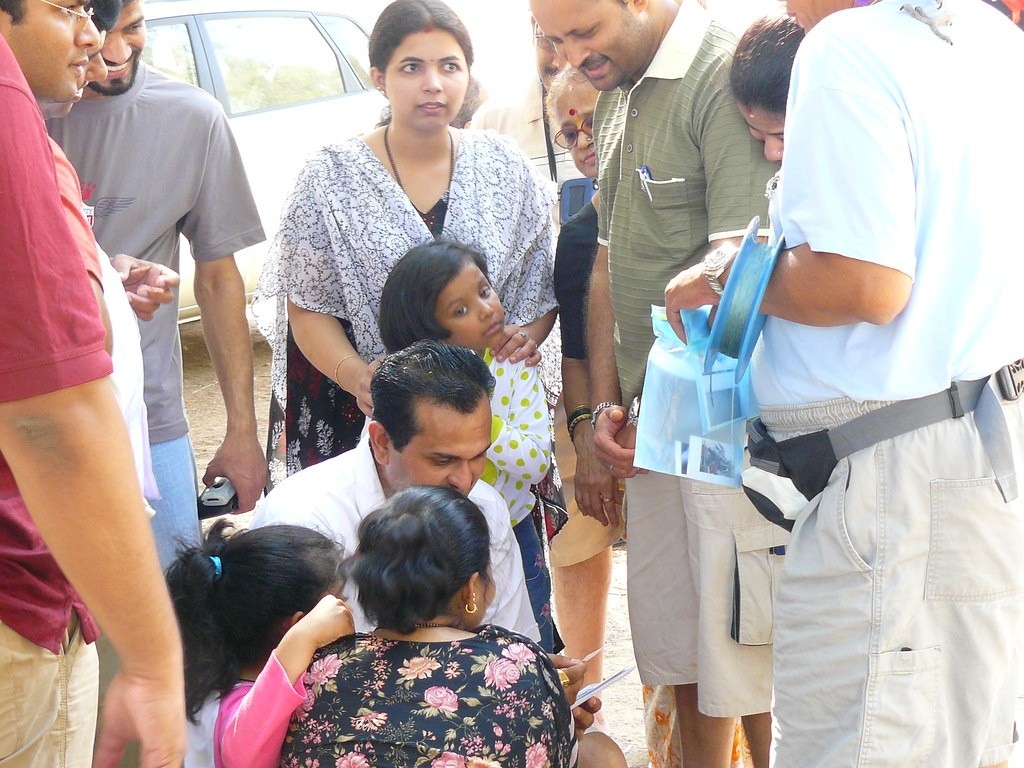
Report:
704,247,737,295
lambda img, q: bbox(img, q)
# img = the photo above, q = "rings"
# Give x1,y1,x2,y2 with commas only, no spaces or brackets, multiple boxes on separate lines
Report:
558,669,570,688
610,465,613,472
519,332,526,339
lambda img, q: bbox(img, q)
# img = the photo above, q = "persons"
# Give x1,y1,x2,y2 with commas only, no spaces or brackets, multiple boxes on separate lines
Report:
246,339,627,768
458,6,603,726
280,485,578,767
246,0,558,480
664,0,1023,768
0,0,266,767
166,519,356,768
530,1,784,768
360,241,555,655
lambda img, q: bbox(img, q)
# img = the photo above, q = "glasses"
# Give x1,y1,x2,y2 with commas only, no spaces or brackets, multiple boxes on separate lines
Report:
553,116,592,149
44,1,94,31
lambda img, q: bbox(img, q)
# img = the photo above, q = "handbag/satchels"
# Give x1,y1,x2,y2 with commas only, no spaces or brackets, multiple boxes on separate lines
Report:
632,303,751,489
741,357,1024,533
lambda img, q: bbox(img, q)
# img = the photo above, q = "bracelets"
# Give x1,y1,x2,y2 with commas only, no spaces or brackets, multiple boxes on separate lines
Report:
627,397,640,427
335,356,360,390
567,404,592,433
591,402,618,430
603,498,610,503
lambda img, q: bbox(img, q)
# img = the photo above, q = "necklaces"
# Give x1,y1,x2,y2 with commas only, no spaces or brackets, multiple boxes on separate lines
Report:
415,623,445,627
385,126,455,190
239,679,255,683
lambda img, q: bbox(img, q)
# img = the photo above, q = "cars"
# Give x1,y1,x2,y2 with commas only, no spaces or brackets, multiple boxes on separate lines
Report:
133,3,391,329
